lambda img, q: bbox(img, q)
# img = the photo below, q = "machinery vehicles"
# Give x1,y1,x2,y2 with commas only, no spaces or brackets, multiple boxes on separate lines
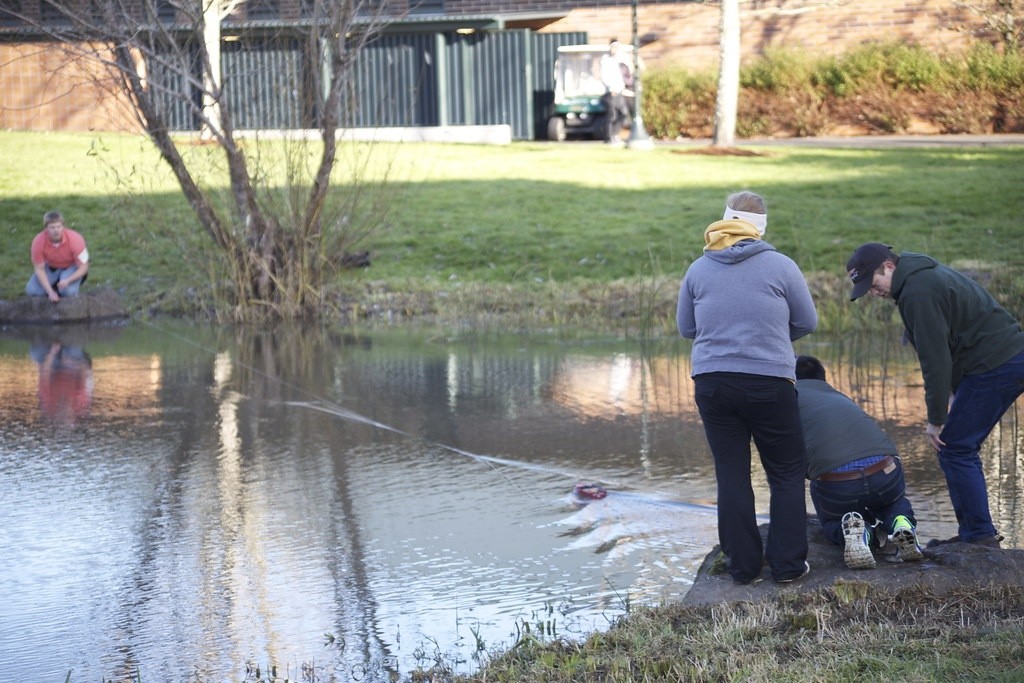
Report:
546,42,629,142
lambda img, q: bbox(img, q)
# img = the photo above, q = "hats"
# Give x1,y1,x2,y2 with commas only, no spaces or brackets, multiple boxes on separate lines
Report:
846,242,893,301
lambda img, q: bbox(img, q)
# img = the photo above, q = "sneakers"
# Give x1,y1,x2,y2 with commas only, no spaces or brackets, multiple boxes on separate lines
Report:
842,511,877,570
890,516,922,561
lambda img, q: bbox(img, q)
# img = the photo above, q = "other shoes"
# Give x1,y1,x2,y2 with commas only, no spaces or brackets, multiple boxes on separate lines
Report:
927,530,1005,549
775,560,811,581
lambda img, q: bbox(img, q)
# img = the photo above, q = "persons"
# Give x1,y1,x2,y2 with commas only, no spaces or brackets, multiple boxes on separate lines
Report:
31,335,94,428
600,39,630,144
848,242,1024,548
676,192,819,586
24,213,88,301
795,355,923,568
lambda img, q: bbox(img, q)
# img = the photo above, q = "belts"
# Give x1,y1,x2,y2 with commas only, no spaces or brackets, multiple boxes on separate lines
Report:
816,455,895,481
